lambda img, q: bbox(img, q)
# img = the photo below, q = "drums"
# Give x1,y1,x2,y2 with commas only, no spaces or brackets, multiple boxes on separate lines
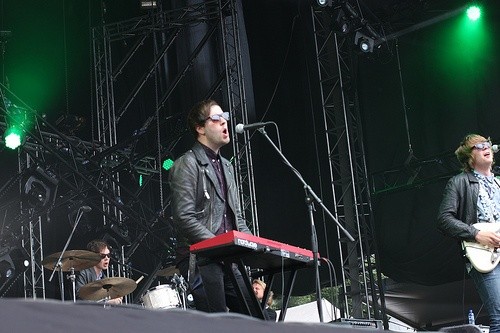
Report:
139,283,184,311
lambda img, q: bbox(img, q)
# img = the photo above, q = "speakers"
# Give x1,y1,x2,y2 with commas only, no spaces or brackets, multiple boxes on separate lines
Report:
439,324,484,333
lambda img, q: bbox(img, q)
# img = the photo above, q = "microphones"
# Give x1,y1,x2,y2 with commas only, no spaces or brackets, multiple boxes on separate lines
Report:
235,121,274,134
80,206,92,213
491,145,500,153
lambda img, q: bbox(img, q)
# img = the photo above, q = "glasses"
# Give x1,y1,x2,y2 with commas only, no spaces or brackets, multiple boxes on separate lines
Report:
471,142,491,150
100,254,110,259
200,112,230,123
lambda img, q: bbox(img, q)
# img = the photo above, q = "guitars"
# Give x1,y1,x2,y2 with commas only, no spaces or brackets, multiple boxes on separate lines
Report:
459,221,500,274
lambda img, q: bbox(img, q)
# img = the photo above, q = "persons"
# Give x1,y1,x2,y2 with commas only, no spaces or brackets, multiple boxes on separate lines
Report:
74,240,123,304
436,134,500,333
251,279,276,322
171,101,264,318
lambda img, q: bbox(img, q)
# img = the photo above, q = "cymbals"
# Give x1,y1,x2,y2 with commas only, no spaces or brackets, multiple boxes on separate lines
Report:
158,264,181,276
79,276,138,300
42,249,102,273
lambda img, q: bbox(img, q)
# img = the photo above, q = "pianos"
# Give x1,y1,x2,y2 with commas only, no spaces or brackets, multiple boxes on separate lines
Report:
189,228,322,275
